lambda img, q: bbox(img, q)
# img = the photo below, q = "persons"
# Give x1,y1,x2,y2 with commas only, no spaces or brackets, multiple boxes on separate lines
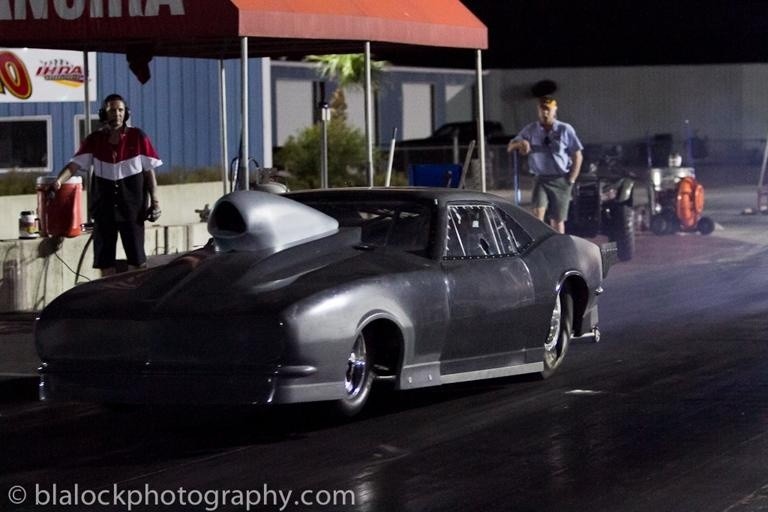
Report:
53,94,164,278
506,96,585,235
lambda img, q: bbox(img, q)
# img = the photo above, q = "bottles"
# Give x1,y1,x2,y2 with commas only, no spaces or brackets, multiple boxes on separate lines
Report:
17,209,34,241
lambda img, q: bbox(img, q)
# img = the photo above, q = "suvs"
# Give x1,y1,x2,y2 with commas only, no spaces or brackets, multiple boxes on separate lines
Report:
563,158,657,263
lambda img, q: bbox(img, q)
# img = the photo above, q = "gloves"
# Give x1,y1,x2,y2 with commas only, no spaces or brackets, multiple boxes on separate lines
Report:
146,205,162,222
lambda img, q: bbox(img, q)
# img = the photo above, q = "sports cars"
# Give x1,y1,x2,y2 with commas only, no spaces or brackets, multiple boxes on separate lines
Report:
29,182,612,426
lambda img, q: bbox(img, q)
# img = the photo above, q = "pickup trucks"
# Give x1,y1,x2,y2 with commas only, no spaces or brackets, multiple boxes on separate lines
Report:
391,118,514,183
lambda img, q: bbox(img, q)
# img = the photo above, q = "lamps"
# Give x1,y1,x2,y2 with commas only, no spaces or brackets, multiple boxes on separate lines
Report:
317,99,333,189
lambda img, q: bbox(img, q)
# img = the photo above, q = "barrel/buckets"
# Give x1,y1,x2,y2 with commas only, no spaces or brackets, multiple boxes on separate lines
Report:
35,175,84,238
653,166,698,228
408,162,462,188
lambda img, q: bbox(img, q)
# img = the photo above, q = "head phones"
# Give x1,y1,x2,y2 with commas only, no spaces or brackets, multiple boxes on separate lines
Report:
98,94,131,122
